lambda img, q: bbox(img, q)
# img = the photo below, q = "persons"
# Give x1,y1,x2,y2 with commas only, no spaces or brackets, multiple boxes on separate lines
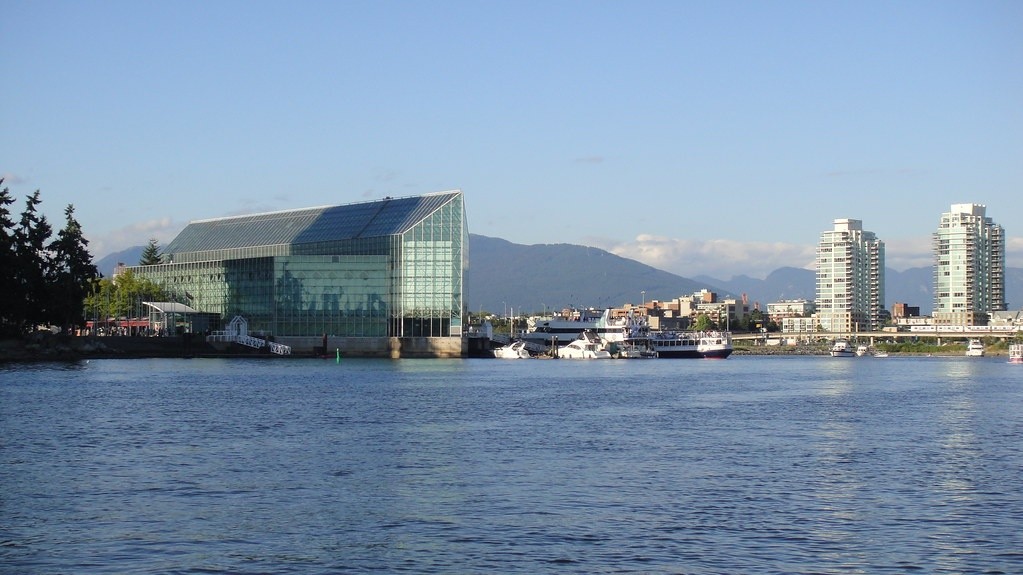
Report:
97,325,128,336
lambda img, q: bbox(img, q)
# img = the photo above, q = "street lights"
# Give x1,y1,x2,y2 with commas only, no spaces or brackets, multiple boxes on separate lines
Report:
640,291,648,308
542,303,546,317
479,304,482,324
503,301,507,317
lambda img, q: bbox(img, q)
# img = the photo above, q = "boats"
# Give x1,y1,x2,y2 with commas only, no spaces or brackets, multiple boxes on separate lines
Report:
855,345,871,357
964,339,986,358
874,350,889,358
828,341,855,357
493,327,735,360
1006,343,1023,364
